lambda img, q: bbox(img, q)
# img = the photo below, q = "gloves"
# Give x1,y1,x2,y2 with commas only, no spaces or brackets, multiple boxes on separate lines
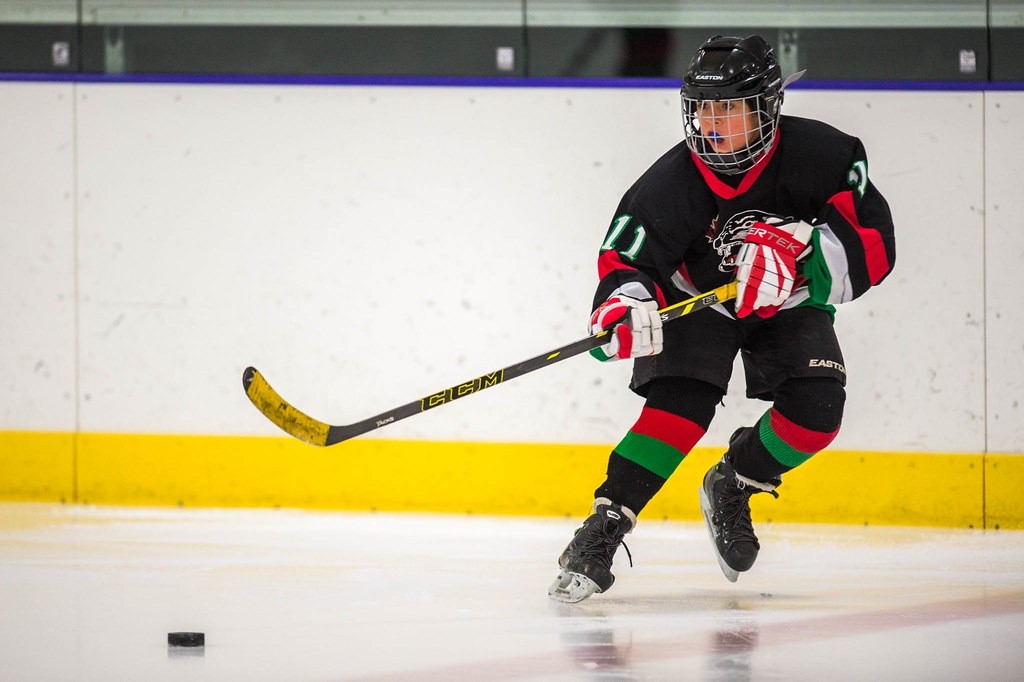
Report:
586,281,665,362
730,217,813,320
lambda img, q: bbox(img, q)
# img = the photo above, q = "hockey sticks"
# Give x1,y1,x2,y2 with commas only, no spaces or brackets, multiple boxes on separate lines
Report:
241,276,740,451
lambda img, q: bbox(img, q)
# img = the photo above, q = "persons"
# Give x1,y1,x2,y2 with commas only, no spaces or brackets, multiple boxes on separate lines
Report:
546,34,899,605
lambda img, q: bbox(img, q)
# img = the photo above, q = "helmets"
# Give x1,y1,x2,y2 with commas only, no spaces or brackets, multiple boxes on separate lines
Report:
684,35,783,118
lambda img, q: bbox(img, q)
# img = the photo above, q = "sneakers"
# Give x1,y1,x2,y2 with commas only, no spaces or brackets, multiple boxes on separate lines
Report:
699,428,781,582
546,496,638,601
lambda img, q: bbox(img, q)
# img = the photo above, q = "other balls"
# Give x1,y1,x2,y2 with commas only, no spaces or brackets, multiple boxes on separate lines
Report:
167,631,205,648
